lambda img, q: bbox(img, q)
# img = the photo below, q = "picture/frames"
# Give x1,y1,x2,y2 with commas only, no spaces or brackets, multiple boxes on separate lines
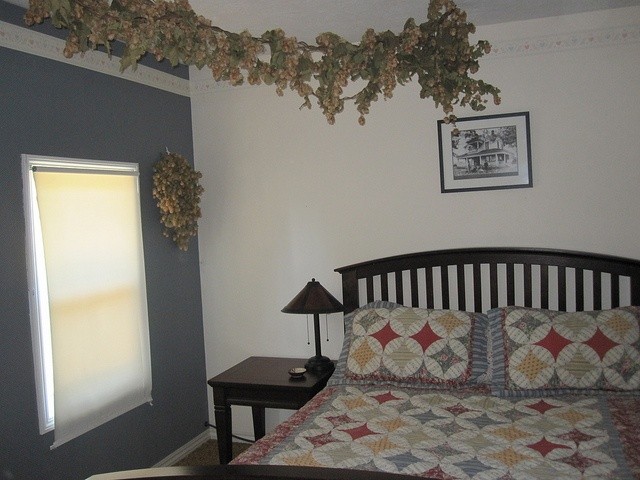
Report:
436,110,533,194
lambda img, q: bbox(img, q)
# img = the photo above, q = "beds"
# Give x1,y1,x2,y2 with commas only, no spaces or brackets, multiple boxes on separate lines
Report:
229,244,639,479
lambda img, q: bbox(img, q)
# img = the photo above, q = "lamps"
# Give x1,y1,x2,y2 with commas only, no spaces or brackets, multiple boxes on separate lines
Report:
281,275,346,375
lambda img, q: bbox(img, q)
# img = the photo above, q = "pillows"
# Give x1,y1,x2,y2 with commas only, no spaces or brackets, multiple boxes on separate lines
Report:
342,300,487,395
487,305,639,399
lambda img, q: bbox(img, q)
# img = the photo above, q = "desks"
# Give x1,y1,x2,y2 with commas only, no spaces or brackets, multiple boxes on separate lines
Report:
206,356,339,466
